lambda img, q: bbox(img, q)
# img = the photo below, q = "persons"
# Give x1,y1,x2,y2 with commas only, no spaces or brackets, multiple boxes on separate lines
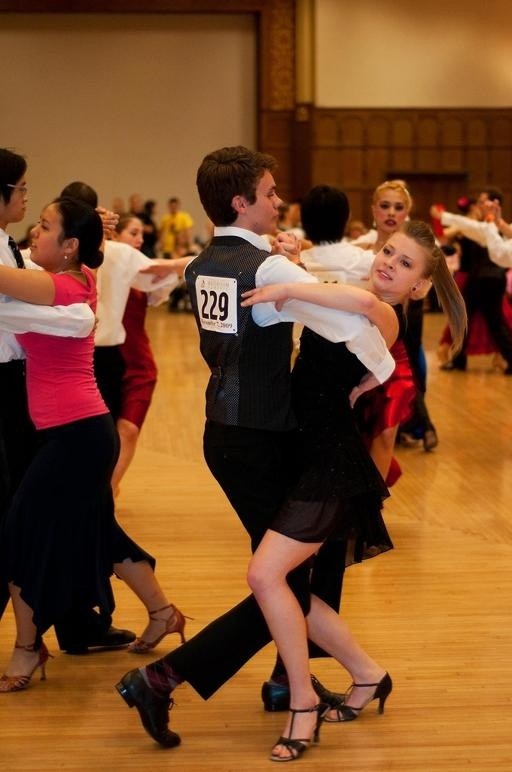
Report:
114,148,467,762
0,147,214,693
422,190,512,376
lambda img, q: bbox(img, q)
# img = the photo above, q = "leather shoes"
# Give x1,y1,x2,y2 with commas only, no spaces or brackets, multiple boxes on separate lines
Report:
66,624,136,656
114,668,181,748
262,674,349,708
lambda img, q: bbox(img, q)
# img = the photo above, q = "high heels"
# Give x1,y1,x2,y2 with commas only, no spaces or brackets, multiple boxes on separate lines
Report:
271,697,326,759
0,644,49,693
326,670,392,721
128,603,189,655
